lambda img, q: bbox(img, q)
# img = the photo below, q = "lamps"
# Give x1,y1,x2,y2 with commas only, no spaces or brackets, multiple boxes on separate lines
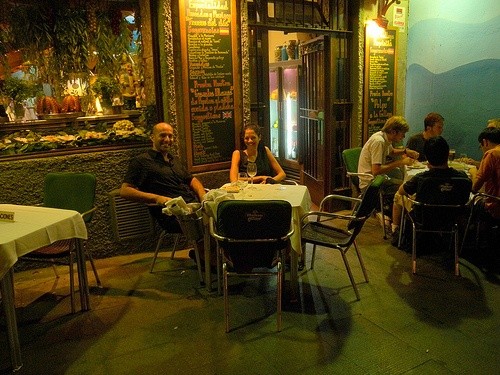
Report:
367,13,390,41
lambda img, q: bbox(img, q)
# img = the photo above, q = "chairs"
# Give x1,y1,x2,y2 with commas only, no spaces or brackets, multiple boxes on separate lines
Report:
206,199,296,333
10,171,105,316
397,194,473,277
460,192,500,255
144,201,185,273
340,146,393,230
296,175,385,302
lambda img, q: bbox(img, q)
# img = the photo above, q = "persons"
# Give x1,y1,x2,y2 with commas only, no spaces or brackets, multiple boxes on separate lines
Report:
404,113,455,161
460,126,500,238
487,118,500,129
230,124,288,269
357,115,414,229
391,135,468,247
120,122,235,275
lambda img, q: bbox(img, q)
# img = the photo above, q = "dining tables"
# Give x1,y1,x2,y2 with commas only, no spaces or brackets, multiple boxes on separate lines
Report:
199,182,310,293
399,160,479,186
0,204,89,374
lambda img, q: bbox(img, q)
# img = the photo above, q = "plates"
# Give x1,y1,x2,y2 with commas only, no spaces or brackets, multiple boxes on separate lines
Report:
408,166,425,168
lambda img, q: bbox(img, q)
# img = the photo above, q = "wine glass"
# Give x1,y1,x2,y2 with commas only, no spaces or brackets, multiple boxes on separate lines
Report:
448,149,456,166
247,163,257,190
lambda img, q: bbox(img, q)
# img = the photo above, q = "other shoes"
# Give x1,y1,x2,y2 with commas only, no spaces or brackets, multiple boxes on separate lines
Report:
375,210,392,236
391,228,403,246
189,249,218,274
